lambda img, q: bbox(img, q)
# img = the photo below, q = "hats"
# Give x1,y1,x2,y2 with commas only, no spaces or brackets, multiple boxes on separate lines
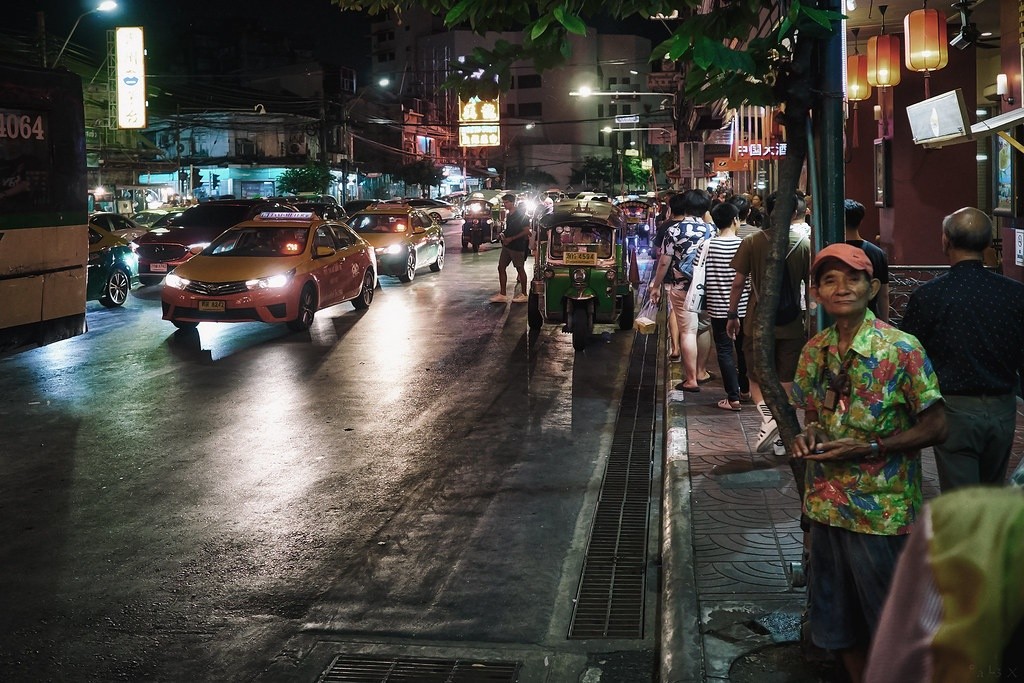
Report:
811,243,873,278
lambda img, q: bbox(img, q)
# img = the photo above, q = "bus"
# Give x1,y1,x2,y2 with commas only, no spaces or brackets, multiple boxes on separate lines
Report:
0,64,87,360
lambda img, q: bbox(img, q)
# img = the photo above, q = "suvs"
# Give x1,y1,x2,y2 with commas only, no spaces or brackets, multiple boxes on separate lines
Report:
129,199,299,286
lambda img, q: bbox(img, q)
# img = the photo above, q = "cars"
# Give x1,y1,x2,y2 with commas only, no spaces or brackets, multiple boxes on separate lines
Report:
88,208,188,308
161,212,378,331
340,204,446,283
275,191,460,235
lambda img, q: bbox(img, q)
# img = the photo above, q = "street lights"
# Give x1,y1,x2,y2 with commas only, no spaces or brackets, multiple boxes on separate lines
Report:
341,75,389,162
502,123,533,189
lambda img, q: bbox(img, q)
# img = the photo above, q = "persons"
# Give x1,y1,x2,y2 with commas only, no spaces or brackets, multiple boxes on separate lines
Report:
691,203,751,411
862,485,1024,683
845,199,891,324
488,194,532,303
651,194,687,364
649,189,719,393
726,189,810,456
787,242,948,683
541,191,553,207
654,189,684,232
789,188,813,344
162,193,200,208
901,206,1024,497
706,179,770,242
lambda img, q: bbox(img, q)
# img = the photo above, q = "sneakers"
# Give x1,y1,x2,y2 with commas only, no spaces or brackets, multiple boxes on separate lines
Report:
756,417,779,453
772,436,785,456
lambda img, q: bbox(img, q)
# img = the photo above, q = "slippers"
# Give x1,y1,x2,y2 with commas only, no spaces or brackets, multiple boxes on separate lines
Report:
669,352,680,361
697,371,715,383
675,379,700,393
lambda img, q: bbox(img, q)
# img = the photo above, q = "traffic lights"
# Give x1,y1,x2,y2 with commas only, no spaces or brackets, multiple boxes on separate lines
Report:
193,168,203,189
213,174,220,190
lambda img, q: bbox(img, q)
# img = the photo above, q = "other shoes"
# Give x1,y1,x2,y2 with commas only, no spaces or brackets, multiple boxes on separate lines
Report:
740,391,752,401
717,398,741,410
488,291,508,303
512,293,528,303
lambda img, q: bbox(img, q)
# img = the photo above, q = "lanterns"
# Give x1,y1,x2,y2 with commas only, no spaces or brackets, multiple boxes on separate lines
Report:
903,8,949,100
847,53,872,149
865,35,901,137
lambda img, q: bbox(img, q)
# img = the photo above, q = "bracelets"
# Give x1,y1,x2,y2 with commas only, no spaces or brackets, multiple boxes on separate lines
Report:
877,436,887,454
869,439,879,455
727,310,738,320
803,421,824,431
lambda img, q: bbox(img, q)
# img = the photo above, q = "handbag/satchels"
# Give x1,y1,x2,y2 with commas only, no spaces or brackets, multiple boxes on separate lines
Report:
634,298,657,335
680,222,711,278
683,237,705,314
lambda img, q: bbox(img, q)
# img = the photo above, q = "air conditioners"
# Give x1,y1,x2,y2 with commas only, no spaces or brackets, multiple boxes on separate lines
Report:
241,143,257,155
287,143,307,154
334,153,344,163
176,140,193,157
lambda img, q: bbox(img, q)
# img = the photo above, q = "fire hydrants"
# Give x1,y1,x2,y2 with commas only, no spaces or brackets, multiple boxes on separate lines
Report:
788,515,811,644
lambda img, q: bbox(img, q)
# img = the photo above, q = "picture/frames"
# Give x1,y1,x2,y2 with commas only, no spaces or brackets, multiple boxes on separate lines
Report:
872,136,888,209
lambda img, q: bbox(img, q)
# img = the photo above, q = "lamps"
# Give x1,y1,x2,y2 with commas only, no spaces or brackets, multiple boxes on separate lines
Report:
873,104,888,134
995,73,1015,105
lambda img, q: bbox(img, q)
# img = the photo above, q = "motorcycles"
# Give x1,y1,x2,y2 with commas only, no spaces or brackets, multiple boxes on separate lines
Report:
462,189,652,351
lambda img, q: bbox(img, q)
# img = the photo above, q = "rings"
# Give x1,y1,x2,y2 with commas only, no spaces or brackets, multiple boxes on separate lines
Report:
795,434,805,438
